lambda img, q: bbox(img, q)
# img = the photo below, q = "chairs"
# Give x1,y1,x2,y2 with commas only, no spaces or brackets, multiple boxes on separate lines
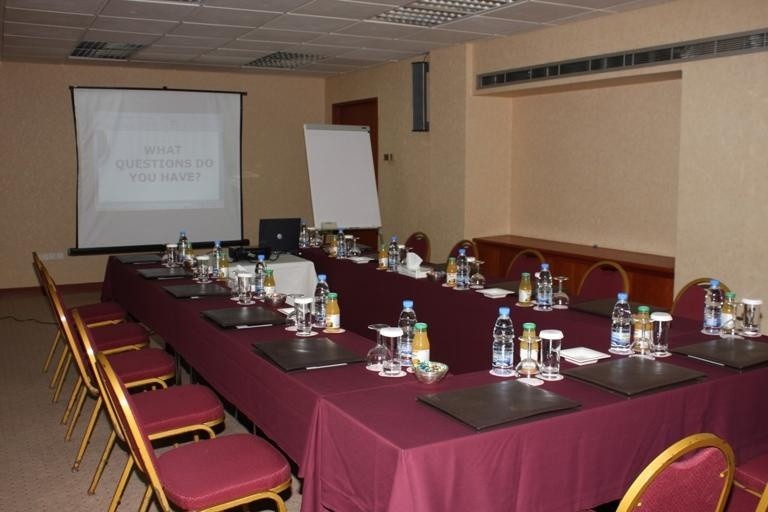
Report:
404,231,432,263
575,261,630,301
668,278,733,322
614,433,735,512
446,240,479,270
505,249,545,286
724,451,768,512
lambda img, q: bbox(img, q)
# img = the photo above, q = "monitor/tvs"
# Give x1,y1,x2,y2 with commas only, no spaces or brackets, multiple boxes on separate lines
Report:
260,219,301,252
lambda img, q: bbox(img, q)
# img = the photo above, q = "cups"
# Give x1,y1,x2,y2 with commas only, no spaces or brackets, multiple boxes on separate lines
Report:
166,227,763,378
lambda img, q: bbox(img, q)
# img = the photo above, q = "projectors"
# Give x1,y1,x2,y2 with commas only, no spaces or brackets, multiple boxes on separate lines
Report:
229,247,270,261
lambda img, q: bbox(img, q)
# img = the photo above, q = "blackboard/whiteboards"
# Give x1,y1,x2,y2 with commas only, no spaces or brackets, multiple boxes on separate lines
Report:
303,122,382,230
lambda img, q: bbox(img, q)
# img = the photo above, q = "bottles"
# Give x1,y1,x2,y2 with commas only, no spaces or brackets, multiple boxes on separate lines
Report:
179,221,736,375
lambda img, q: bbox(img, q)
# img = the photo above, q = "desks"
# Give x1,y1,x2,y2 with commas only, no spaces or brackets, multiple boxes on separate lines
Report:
191,253,318,307
185,306,455,479
301,331,767,512
298,247,640,375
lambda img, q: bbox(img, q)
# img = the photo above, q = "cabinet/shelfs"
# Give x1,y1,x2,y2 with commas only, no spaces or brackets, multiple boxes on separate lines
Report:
473,234,675,309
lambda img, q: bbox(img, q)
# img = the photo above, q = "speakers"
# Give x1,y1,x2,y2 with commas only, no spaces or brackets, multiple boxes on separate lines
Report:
410,61,429,133
